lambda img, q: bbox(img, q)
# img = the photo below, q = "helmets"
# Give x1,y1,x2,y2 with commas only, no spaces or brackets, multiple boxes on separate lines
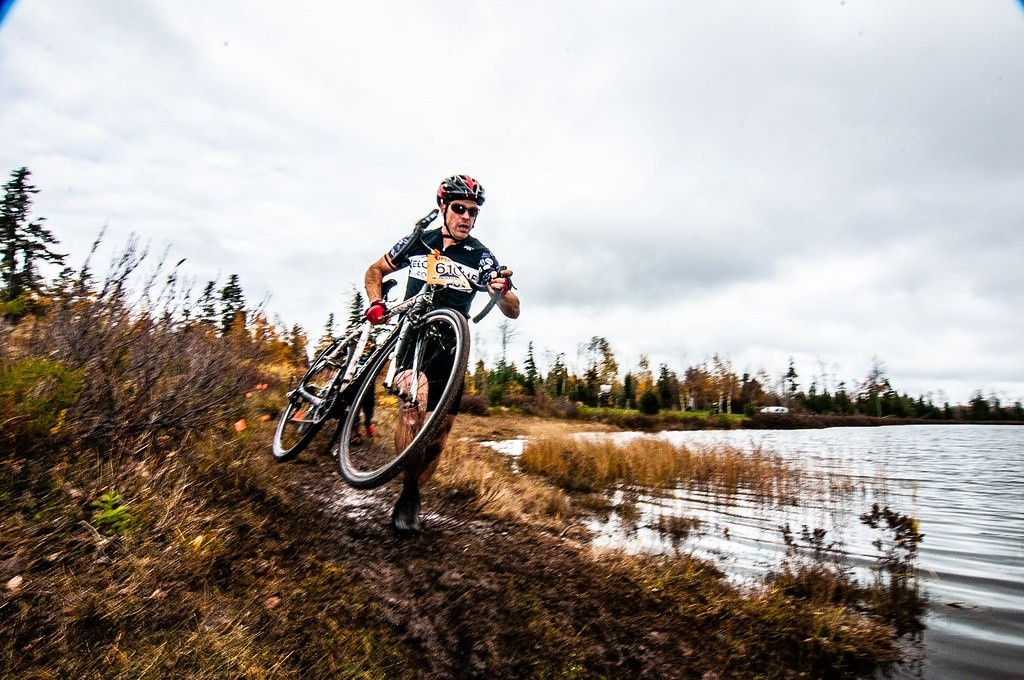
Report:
437,174,486,207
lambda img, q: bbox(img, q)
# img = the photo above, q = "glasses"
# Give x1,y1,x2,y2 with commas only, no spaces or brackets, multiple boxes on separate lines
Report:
448,202,479,217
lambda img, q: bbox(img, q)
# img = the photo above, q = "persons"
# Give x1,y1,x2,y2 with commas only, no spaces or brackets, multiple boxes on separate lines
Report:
365,175,520,532
346,330,381,445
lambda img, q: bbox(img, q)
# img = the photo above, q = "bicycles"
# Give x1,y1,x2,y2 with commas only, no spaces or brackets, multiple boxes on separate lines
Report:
273,210,518,491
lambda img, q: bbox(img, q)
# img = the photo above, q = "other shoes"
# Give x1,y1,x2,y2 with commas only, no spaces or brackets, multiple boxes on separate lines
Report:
365,424,376,438
392,497,421,536
353,434,362,444
